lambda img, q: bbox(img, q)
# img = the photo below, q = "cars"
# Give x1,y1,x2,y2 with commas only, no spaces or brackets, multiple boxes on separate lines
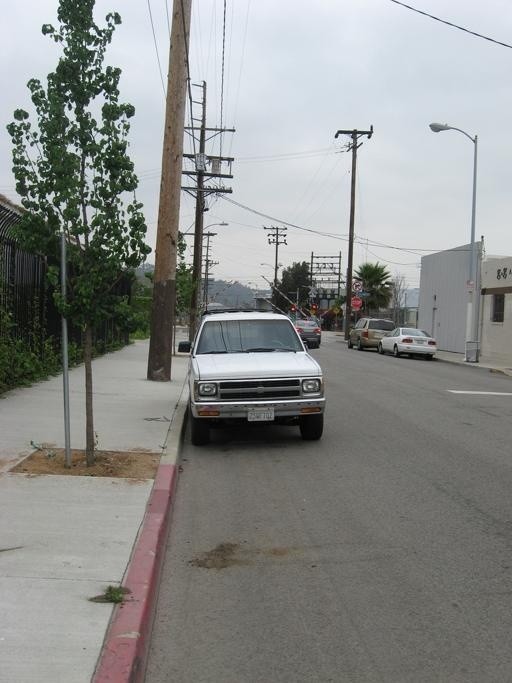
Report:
179,308,326,445
348,318,437,360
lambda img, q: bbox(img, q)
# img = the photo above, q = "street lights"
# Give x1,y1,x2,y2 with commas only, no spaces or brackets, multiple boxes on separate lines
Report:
430,122,481,362
